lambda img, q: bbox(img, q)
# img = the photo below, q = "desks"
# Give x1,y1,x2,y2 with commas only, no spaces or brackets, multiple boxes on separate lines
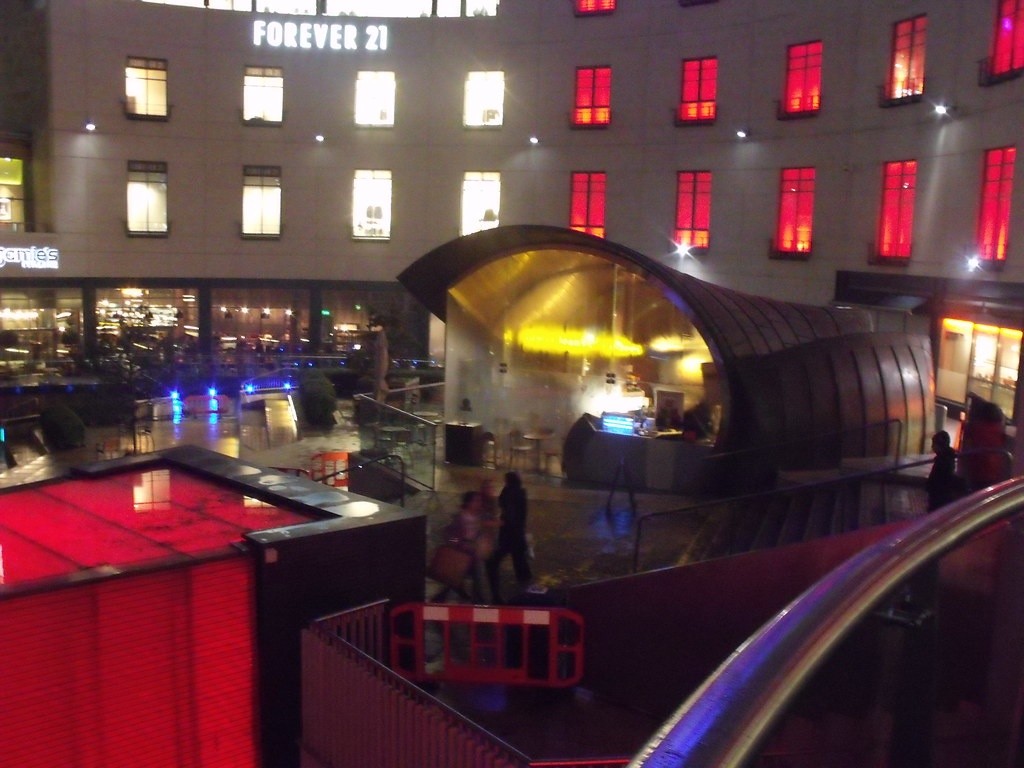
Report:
523,432,555,467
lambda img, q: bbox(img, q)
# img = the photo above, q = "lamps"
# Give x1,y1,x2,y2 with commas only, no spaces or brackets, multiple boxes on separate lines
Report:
935,102,958,114
735,128,752,138
529,135,539,144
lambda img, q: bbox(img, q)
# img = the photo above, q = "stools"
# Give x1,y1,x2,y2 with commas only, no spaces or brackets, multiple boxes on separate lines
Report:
545,449,562,471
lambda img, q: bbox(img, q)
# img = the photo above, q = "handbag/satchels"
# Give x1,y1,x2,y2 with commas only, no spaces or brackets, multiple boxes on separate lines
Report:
524,534,534,561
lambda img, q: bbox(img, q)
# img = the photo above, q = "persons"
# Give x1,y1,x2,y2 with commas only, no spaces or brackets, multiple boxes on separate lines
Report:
927,431,955,510
430,479,504,603
684,403,713,439
657,396,682,430
488,472,533,603
966,405,1009,489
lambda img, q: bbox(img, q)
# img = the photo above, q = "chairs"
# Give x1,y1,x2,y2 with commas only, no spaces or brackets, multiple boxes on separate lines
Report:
95,437,121,461
508,430,533,471
481,431,507,471
